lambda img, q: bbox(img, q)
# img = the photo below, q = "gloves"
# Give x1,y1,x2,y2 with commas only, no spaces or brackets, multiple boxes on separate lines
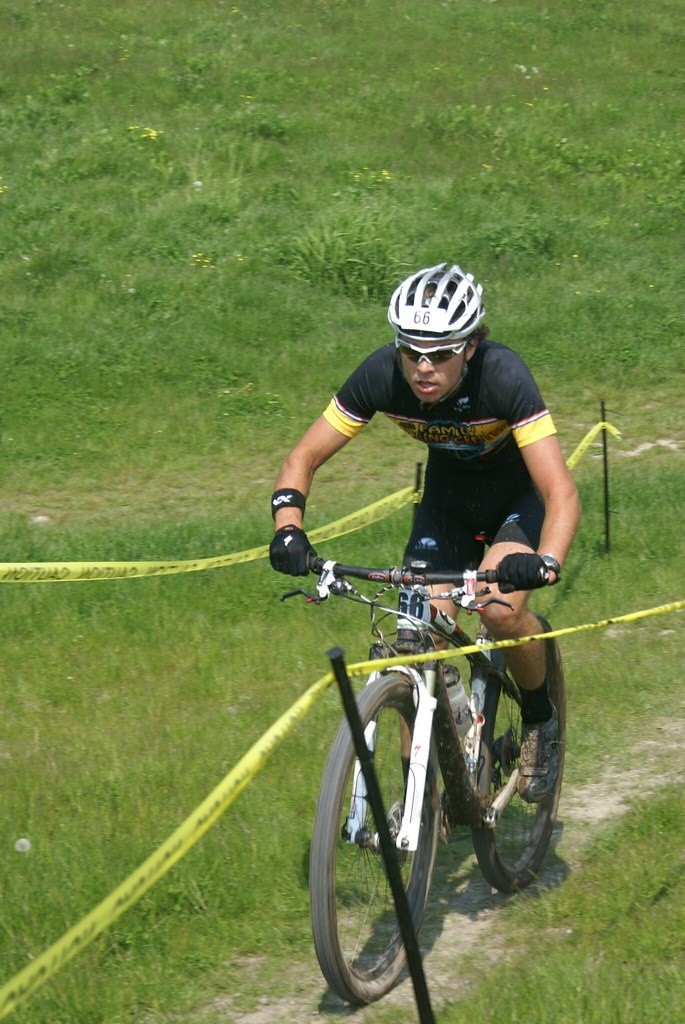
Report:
269,525,317,576
496,552,550,593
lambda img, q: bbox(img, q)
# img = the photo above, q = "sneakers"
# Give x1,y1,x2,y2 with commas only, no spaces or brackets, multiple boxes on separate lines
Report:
516,698,560,803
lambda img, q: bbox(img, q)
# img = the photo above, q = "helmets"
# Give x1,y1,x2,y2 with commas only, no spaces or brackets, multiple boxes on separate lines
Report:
386,262,485,340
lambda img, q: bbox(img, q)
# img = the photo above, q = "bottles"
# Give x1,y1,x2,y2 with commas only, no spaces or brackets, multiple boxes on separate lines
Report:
440,665,473,736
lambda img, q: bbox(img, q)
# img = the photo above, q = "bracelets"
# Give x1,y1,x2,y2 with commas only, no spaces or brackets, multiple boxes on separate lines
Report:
270,489,306,521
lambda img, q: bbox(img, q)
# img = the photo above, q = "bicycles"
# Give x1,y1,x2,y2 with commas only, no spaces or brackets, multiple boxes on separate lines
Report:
283,554,571,1007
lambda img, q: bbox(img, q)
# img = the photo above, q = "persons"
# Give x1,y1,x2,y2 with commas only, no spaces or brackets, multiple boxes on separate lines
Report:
270,263,581,849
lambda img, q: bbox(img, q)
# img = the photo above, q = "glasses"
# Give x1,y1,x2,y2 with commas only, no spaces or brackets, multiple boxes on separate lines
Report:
394,332,467,365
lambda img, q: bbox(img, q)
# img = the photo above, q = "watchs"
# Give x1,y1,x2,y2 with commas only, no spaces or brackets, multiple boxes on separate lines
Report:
540,553,561,574
